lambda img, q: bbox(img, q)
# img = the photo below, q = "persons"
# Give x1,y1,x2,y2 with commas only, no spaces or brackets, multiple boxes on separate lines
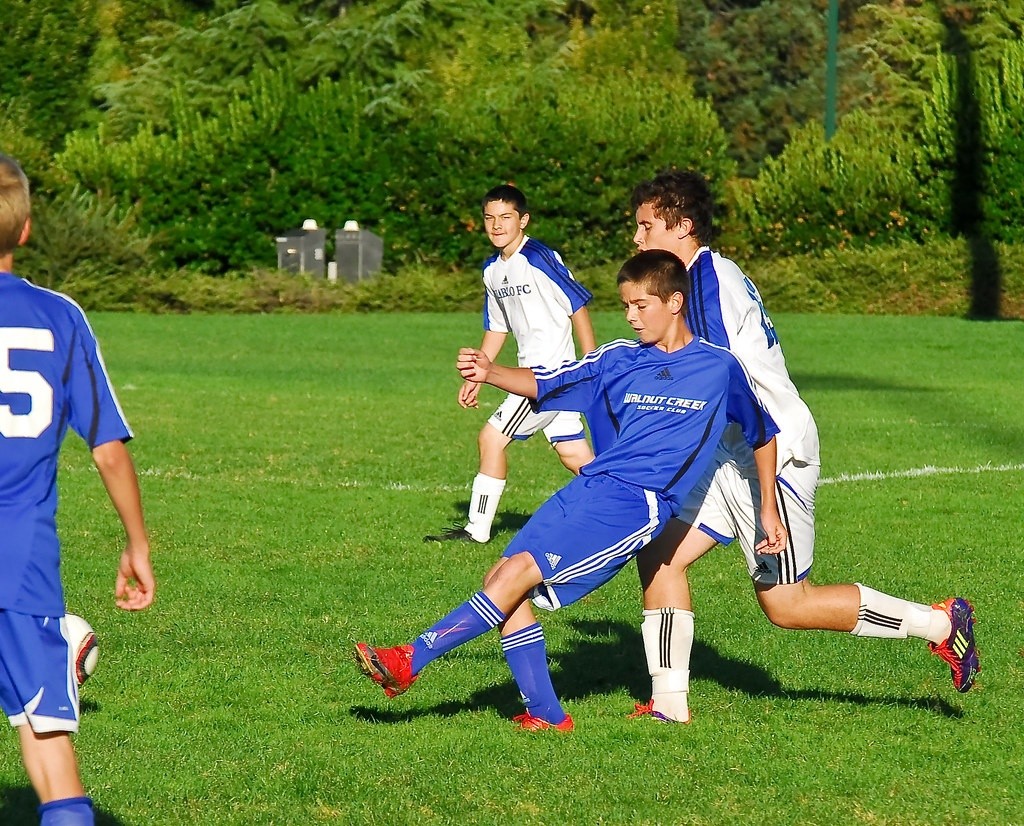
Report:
422,185,597,545
627,172,983,723
356,249,788,732
0,154,155,826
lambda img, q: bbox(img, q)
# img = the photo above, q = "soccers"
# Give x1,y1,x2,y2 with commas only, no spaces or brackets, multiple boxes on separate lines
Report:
60,613,99,687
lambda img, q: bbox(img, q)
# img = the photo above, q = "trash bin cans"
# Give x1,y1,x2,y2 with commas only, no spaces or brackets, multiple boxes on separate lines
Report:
336,219,384,283
274,218,328,280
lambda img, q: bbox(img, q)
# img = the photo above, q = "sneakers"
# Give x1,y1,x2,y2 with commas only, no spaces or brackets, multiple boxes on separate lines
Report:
422,521,491,545
926,597,981,693
512,707,574,732
625,699,691,725
351,643,418,699
